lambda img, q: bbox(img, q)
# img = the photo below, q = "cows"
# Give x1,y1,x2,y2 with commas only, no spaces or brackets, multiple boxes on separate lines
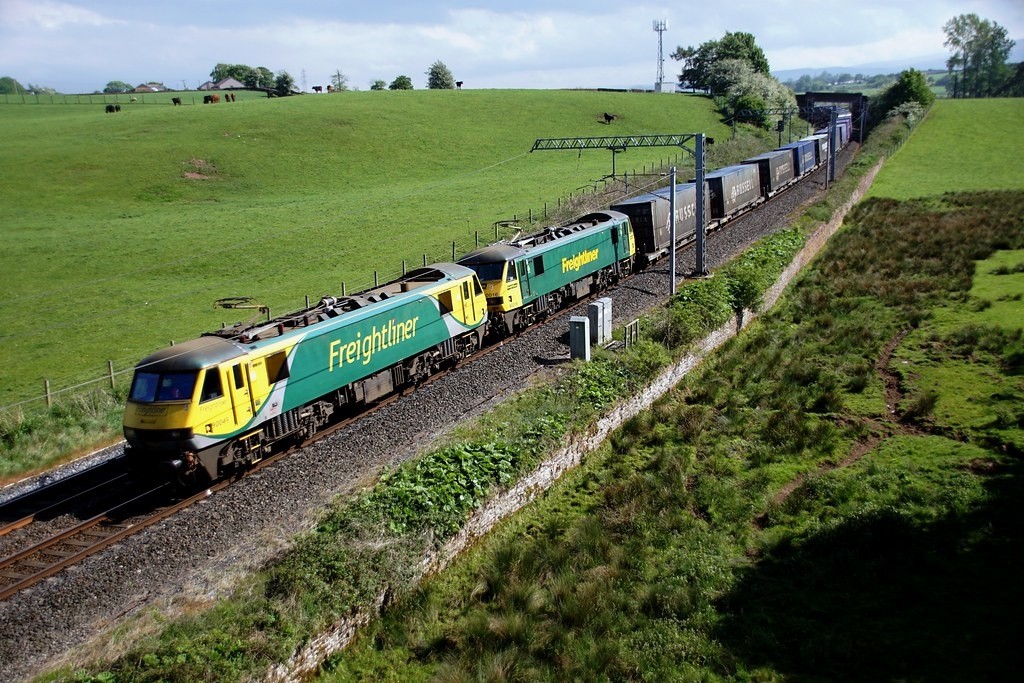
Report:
203,93,220,103
312,86,322,93
706,137,714,145
106,105,120,113
130,97,137,103
224,93,235,102
603,113,616,124
327,85,332,93
267,91,275,98
172,97,181,105
456,81,463,89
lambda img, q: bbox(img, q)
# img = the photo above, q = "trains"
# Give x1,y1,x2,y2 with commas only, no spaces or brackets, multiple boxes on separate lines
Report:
121,107,853,473
